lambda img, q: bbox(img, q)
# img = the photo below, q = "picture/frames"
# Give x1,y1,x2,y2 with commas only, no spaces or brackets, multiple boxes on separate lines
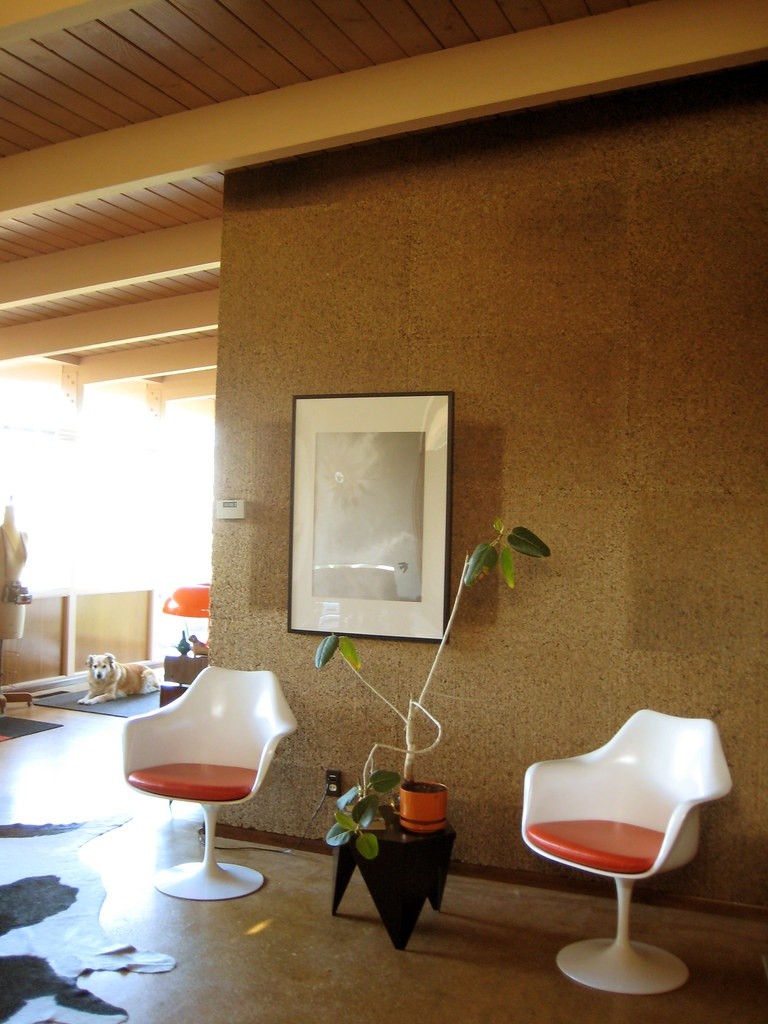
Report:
286,388,454,644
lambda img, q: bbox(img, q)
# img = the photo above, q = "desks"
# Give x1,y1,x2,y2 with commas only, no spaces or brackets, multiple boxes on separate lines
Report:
332,814,456,950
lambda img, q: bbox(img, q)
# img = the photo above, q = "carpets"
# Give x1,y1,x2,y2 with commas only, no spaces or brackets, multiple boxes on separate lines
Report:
33,690,161,718
0,716,65,742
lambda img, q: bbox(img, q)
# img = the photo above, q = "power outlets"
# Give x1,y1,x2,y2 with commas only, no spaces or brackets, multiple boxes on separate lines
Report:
325,770,342,797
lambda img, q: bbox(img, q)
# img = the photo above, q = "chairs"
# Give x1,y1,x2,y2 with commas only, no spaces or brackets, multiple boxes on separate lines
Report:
520,708,733,996
121,665,299,901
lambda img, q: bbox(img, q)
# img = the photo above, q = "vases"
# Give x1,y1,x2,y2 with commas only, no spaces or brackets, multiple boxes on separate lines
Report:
398,781,448,833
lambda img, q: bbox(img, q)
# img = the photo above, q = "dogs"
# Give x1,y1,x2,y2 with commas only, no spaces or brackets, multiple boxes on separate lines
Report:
77,653,160,705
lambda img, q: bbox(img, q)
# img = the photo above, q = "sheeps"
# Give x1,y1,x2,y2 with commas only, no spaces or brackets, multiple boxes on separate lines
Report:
188,635,209,657
